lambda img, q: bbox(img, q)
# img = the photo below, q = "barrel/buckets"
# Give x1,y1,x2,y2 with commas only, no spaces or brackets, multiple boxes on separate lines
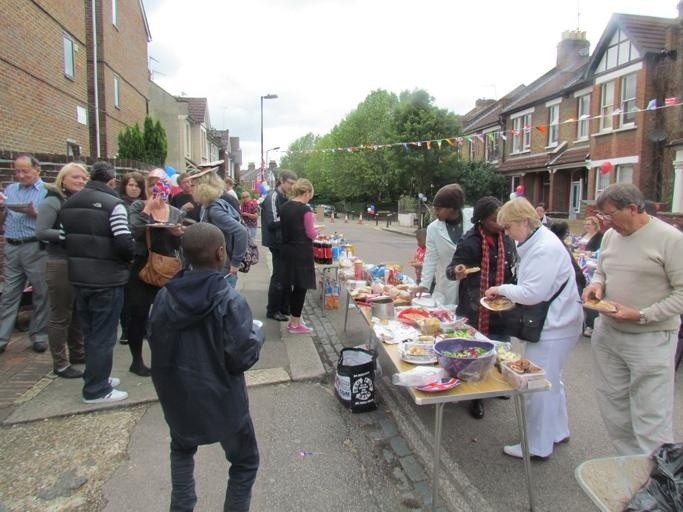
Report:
369,297,395,318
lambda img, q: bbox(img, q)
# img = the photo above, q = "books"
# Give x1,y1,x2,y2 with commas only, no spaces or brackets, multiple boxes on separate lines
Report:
366,204,375,216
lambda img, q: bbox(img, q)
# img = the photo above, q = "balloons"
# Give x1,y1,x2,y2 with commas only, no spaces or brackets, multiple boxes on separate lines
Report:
253,178,272,196
602,161,611,173
510,186,525,200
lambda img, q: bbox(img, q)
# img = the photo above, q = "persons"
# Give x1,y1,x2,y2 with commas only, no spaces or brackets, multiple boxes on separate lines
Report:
484,197,585,461
406,182,476,309
278,176,320,336
258,168,298,322
408,227,427,283
146,222,265,511
1,152,261,405
534,200,661,337
580,182,683,456
444,195,520,420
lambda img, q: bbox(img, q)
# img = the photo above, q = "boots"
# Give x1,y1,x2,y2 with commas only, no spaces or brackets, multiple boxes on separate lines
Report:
120,322,128,344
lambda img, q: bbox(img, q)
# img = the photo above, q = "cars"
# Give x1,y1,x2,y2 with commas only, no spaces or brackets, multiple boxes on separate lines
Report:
307,204,337,217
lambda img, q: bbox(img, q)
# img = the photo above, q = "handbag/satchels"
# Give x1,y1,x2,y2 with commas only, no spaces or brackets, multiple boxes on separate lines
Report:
333,347,378,413
508,299,549,343
138,252,183,286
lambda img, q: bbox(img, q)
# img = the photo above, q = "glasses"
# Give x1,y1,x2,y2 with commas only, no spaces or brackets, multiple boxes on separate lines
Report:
595,211,616,222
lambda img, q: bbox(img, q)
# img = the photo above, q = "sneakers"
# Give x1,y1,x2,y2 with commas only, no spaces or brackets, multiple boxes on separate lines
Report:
287,321,305,328
108,377,120,388
583,326,593,340
288,324,313,334
82,388,128,404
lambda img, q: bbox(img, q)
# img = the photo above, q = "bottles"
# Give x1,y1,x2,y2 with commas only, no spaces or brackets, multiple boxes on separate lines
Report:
313,231,353,265
319,274,339,310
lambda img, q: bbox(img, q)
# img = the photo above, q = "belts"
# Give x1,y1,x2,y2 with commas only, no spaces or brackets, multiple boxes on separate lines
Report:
7,237,37,245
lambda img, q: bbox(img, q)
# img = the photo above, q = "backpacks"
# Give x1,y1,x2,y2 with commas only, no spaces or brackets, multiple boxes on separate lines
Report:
207,203,259,272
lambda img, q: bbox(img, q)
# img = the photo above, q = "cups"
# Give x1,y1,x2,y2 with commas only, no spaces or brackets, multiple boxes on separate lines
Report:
354,259,362,280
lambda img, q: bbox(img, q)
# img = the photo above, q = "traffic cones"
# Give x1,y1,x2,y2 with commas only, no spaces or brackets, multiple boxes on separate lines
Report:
356,212,363,225
343,214,348,224
330,211,334,224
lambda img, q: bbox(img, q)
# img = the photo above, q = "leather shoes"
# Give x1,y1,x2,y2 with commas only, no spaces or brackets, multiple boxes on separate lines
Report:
267,310,288,321
33,341,48,352
54,366,82,378
470,400,484,418
503,442,535,459
129,363,152,376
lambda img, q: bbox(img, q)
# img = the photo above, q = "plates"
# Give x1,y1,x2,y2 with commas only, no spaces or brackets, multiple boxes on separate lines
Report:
145,221,178,229
1,203,30,210
581,303,616,314
347,281,515,393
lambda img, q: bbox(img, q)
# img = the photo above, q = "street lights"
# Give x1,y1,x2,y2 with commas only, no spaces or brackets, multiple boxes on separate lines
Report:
264,146,280,170
259,94,278,199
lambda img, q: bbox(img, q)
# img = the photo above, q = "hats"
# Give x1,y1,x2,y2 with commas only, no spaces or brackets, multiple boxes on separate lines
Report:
432,182,465,208
